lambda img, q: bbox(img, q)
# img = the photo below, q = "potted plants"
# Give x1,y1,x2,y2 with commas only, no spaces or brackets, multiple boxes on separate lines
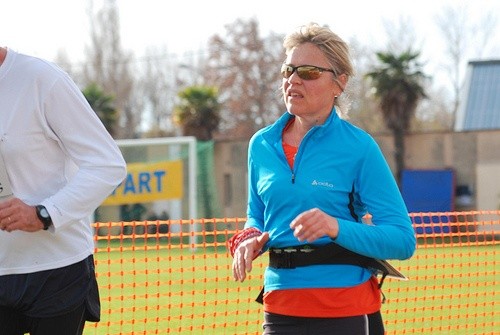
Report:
455,185,474,208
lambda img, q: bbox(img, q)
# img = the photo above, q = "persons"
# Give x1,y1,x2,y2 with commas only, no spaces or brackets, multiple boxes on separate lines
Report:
0,47,126,335
229,23,417,335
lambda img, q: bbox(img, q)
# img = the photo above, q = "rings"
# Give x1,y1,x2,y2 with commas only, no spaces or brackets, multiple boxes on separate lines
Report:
8,217,12,221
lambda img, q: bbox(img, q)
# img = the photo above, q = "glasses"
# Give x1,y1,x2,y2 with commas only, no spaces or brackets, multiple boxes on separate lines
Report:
280,63,337,80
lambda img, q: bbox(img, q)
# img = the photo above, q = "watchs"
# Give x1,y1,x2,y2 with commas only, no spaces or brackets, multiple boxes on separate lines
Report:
34,204,52,230
